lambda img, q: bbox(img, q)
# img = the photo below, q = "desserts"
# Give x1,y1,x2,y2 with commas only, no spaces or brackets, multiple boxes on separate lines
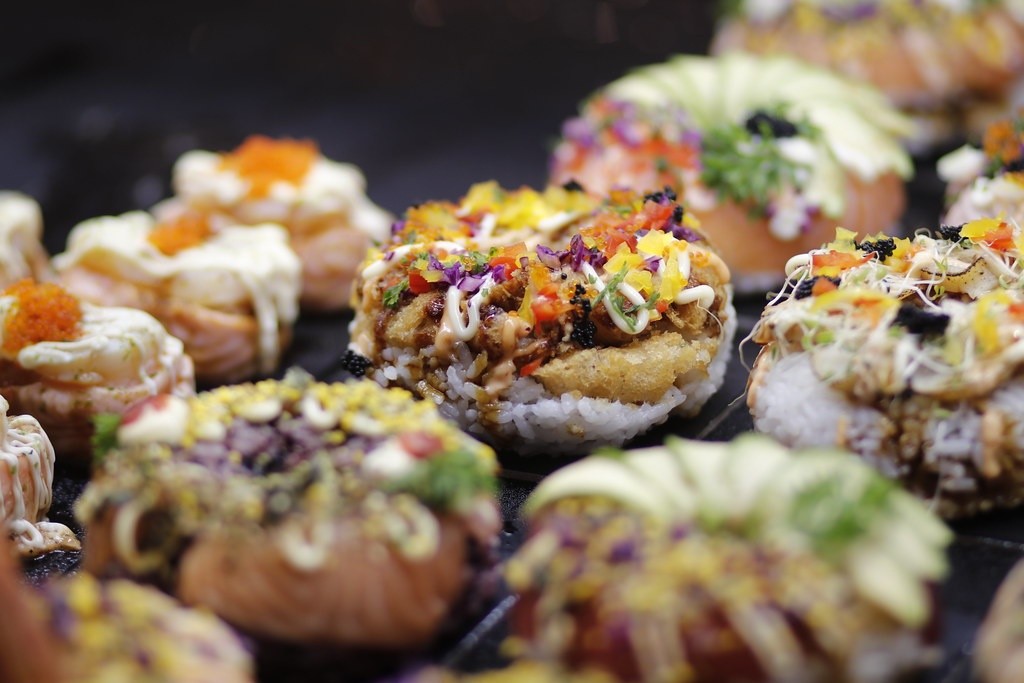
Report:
0,131,385,539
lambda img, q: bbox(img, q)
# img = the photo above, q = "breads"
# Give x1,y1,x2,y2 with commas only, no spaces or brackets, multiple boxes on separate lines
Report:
546,3,1023,288
